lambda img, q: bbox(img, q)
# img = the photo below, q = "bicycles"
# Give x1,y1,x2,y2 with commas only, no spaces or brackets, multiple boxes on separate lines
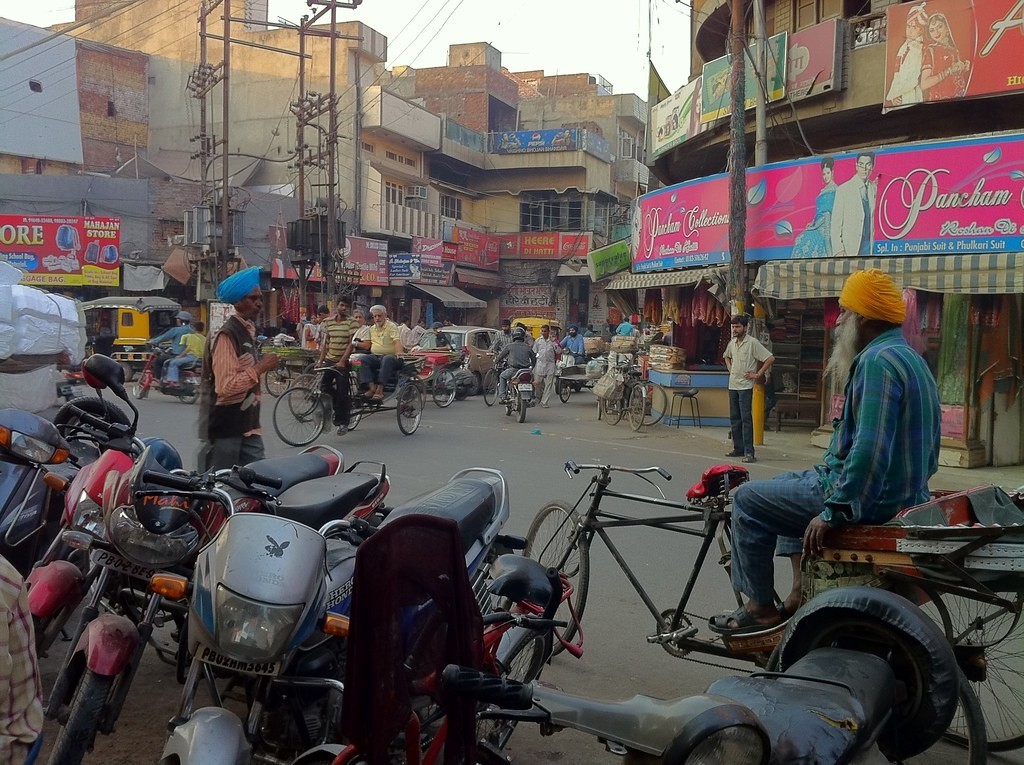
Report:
592,352,667,431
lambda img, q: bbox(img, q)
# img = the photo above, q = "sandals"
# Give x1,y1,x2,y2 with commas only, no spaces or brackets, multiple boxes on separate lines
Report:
742,456,757,462
725,449,744,457
360,391,375,398
372,393,384,399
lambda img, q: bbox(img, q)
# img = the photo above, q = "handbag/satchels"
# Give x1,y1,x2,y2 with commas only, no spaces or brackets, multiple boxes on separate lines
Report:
593,376,624,400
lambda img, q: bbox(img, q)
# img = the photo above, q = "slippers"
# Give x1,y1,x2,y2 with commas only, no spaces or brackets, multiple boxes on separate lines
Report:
708,606,777,634
777,602,792,620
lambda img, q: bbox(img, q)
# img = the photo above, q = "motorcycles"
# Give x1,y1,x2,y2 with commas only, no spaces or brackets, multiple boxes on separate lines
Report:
132,343,202,405
494,359,536,424
83,296,183,382
0,355,959,765
510,317,562,340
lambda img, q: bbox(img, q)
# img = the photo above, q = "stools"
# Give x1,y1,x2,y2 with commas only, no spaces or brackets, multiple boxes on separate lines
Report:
668,389,701,429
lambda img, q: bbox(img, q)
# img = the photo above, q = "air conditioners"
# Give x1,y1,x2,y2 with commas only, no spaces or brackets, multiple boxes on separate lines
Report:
405,185,428,199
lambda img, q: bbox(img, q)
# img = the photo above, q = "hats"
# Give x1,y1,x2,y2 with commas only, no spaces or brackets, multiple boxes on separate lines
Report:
216,266,259,304
838,269,906,323
176,311,190,320
502,319,511,325
569,324,578,331
517,323,527,330
433,322,443,328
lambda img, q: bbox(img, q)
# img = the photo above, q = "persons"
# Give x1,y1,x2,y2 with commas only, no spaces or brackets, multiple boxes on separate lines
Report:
570,299,579,323
708,270,940,632
196,266,282,475
885,1,928,105
353,309,415,357
411,318,432,347
442,319,456,326
351,304,405,404
429,322,456,352
789,152,877,259
723,316,775,463
919,12,970,100
487,317,641,408
301,304,330,398
313,295,359,436
148,311,206,389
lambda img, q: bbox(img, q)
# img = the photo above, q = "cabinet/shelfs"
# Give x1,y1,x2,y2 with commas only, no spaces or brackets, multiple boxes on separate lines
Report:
765,297,829,403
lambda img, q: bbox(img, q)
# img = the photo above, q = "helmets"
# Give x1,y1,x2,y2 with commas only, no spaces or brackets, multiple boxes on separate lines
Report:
512,327,525,339
141,436,183,471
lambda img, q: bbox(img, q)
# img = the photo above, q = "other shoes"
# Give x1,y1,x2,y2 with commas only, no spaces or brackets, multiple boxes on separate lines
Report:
499,399,511,405
540,402,549,408
337,425,348,436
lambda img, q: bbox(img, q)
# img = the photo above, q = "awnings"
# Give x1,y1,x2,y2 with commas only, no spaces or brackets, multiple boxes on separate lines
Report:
753,252,1024,295
409,280,488,308
604,268,704,289
557,263,590,277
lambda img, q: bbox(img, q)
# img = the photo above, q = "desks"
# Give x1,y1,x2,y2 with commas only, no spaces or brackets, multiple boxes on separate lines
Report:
775,400,823,429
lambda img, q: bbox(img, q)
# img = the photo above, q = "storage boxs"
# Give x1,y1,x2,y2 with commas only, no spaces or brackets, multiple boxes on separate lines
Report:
584,335,638,354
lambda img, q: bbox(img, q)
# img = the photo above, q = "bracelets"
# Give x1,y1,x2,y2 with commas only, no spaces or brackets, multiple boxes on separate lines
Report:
939,70,948,80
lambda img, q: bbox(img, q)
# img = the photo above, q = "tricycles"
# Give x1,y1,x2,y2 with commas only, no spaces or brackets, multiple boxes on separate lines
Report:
523,460,1024,764
265,330,605,408
272,366,421,447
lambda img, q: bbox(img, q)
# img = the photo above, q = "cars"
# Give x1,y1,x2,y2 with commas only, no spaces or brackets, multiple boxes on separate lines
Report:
437,325,500,396
